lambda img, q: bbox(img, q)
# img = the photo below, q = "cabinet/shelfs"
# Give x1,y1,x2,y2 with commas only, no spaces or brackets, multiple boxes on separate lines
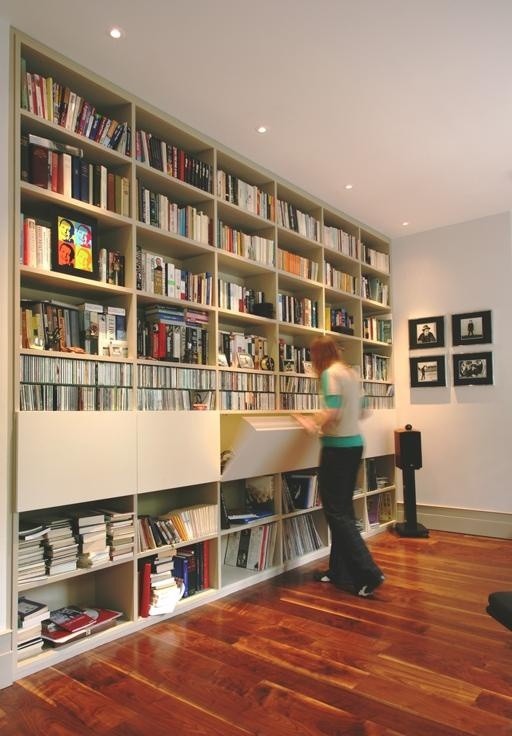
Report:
3,23,397,684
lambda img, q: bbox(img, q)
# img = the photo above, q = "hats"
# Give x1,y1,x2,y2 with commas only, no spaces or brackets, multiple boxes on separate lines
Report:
422,325,430,331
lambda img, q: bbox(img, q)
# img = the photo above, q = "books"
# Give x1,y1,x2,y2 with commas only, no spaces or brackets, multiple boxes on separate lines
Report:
17,41,393,411
15,600,124,660
355,460,393,532
17,506,135,584
219,476,279,572
138,502,218,618
281,472,324,562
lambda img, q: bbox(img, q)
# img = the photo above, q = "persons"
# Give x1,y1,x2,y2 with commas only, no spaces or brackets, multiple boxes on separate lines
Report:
466,319,477,338
421,366,427,381
297,332,383,597
418,325,438,344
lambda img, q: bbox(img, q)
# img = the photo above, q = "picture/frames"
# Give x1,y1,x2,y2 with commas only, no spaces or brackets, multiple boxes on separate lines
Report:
407,309,493,387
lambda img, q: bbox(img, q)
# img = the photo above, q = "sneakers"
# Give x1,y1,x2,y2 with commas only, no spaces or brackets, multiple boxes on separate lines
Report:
315,569,334,582
358,573,384,596
